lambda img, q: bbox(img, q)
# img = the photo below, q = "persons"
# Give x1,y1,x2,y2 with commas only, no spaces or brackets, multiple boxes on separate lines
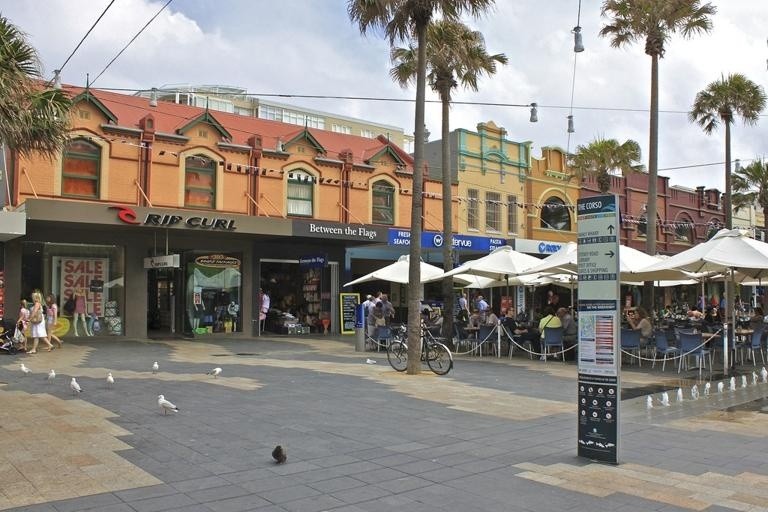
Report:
361,289,768,362
16,288,63,354
72,284,92,337
193,298,206,329
227,301,239,332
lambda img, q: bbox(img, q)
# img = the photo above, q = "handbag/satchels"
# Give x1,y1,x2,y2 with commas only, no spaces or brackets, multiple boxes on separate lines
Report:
457,310,468,321
64,296,75,311
31,310,42,323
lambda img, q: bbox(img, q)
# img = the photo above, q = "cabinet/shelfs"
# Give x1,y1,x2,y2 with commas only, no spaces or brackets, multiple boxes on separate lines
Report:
296,283,321,332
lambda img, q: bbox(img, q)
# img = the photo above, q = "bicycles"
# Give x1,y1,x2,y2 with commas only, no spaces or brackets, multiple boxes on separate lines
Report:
385,319,454,375
0,320,28,355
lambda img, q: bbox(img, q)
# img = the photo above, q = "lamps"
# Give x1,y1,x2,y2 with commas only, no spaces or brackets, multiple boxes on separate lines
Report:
568,115,574,132
530,103,538,121
572,27,584,52
54,69,62,90
150,87,157,106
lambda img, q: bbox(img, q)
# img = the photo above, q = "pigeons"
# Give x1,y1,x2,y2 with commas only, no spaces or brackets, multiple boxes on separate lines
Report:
47,369,56,385
70,377,84,397
151,361,160,374
271,445,287,465
19,364,33,377
157,393,180,417
106,372,115,386
204,366,224,380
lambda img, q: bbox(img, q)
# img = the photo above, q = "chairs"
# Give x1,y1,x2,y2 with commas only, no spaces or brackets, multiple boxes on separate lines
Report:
377,315,579,363
621,315,768,375
